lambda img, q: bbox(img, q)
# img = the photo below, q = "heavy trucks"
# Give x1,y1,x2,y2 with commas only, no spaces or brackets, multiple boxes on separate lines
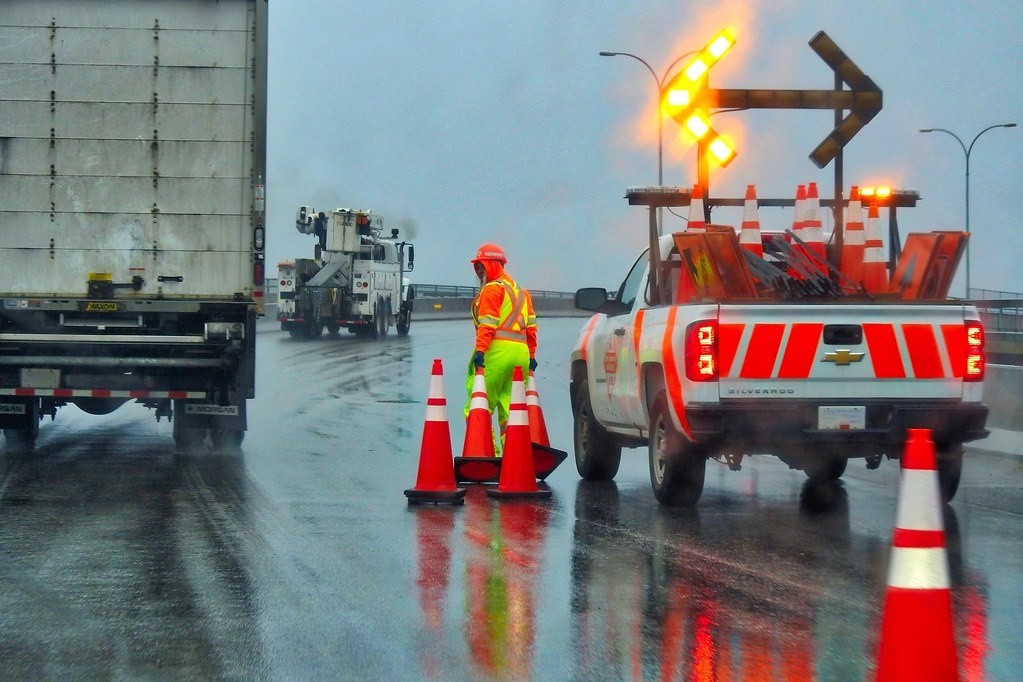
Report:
273,205,415,340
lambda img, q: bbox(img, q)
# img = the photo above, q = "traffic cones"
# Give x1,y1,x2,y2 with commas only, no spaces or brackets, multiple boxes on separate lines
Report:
675,184,706,305
839,185,867,293
738,184,764,283
872,427,962,682
453,364,503,483
847,203,901,294
404,358,468,505
786,182,830,280
525,369,568,482
485,363,552,498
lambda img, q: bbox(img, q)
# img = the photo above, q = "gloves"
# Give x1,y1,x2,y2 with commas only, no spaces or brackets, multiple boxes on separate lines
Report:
529,358,538,372
473,352,485,371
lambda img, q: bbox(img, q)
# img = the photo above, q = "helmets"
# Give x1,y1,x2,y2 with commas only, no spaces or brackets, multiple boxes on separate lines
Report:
470,244,507,264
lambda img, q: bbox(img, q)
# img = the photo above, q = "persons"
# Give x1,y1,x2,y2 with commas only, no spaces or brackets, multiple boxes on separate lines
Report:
462,243,538,459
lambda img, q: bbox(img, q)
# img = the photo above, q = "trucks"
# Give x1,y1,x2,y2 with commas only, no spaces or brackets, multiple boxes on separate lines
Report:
0,0,271,450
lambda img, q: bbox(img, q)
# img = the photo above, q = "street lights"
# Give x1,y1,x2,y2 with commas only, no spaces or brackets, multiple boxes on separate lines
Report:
918,121,1017,303
599,39,738,238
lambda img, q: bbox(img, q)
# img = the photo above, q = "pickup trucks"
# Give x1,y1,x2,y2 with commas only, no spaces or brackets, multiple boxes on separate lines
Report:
569,181,993,506
